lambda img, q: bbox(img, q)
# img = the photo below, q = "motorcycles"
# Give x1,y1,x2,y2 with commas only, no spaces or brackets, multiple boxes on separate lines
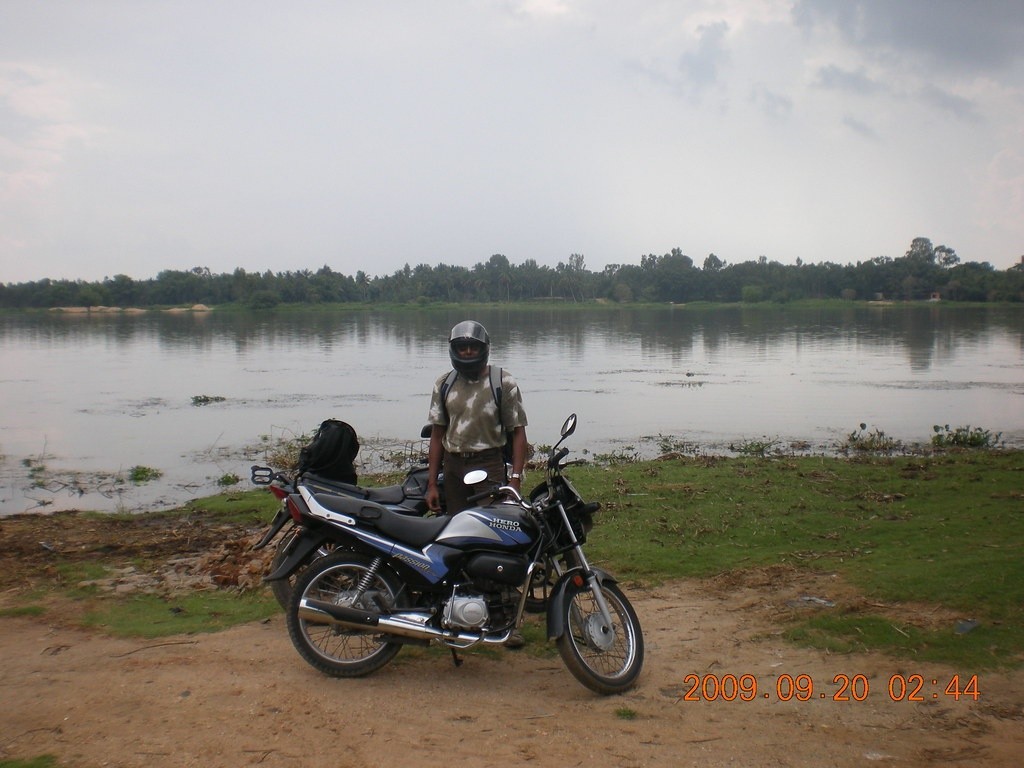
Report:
247,411,648,697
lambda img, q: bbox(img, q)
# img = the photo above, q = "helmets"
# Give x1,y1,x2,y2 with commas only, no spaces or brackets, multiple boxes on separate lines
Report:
449,320,490,372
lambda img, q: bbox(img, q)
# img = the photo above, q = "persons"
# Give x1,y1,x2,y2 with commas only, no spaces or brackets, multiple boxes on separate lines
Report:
427,320,529,648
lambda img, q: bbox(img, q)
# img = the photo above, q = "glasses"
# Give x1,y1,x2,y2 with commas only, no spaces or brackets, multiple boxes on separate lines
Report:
453,341,483,352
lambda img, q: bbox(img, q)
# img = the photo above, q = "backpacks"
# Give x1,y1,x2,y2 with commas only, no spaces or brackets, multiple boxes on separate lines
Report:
299,420,359,486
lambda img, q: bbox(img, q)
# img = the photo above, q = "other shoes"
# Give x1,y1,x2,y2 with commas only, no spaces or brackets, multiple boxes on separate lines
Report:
502,627,526,648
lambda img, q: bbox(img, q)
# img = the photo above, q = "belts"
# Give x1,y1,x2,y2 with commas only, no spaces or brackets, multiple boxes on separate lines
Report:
447,452,502,458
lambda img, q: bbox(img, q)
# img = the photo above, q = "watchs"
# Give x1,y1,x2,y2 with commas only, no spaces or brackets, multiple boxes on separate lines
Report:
509,474,524,481
